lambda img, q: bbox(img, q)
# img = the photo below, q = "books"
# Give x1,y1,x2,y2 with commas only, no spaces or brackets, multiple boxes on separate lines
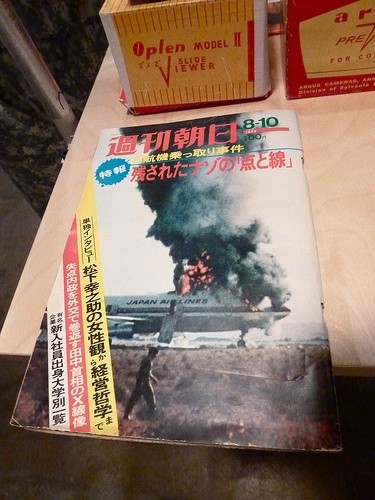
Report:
10,109,343,452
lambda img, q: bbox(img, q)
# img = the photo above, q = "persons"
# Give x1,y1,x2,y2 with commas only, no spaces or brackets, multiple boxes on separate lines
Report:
122,347,160,420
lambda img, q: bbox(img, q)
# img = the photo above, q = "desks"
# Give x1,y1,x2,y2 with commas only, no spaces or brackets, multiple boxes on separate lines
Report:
0,7,373,405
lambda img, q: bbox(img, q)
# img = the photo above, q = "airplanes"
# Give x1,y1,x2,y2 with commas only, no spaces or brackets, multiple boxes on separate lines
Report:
99,287,290,339
